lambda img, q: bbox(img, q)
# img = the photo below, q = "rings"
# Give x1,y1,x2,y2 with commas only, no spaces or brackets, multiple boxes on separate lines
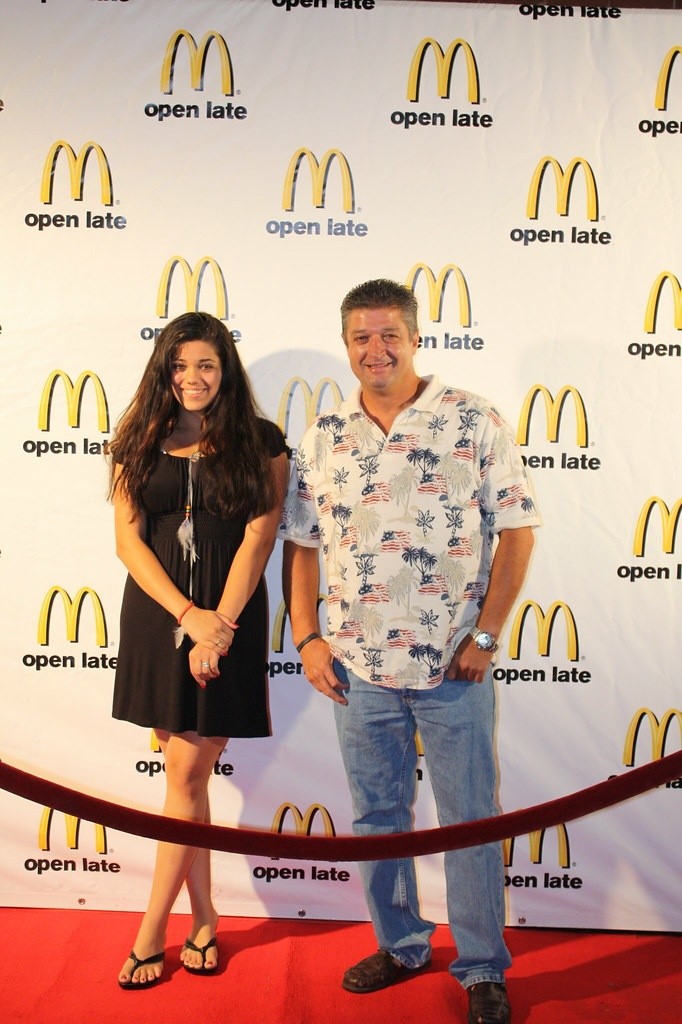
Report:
216,639,223,645
201,662,210,666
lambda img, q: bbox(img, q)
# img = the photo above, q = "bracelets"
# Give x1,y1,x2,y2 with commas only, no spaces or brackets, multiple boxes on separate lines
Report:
296,633,320,653
177,600,195,625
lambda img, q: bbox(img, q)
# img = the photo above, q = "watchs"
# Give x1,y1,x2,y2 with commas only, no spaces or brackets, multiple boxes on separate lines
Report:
469,626,499,653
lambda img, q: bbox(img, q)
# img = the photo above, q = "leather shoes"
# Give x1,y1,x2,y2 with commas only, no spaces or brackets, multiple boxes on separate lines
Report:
467,982,511,1024
342,950,432,992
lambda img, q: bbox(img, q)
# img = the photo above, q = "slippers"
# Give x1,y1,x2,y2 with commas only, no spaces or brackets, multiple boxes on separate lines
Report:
118,949,165,990
182,936,218,974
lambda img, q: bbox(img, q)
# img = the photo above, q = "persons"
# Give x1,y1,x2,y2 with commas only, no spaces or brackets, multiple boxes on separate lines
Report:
276,279,542,1024
109,312,292,990
